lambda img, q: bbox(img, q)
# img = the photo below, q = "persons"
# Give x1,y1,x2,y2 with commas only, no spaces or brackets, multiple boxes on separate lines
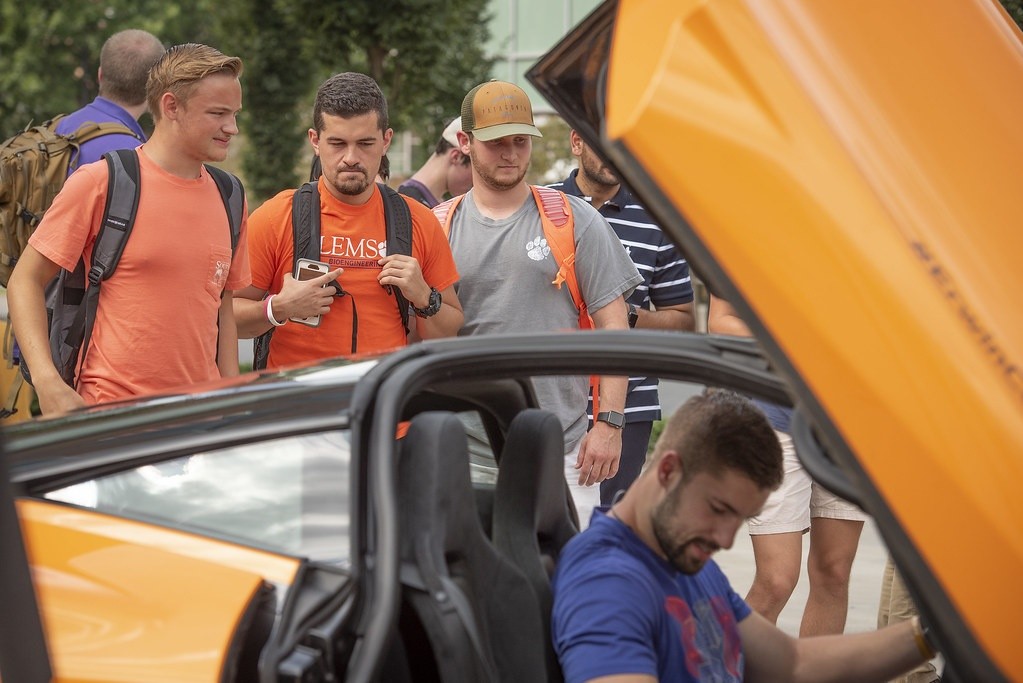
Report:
0,29,937,683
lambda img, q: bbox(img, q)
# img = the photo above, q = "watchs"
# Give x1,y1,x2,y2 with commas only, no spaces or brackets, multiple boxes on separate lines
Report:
595,409,626,433
409,285,442,319
626,304,639,328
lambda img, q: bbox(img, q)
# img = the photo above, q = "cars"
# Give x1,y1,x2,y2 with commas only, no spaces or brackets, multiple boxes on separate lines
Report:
0,0,1023,682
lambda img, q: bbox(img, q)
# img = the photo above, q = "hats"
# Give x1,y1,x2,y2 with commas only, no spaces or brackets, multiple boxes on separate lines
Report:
441,116,461,149
461,78,544,142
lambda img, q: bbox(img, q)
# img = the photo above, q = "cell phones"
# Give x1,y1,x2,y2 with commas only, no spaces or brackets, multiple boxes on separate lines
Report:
289,257,330,328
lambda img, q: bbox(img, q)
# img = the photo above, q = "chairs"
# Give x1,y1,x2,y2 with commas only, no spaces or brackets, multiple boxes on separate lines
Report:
491,410,581,683
394,410,550,683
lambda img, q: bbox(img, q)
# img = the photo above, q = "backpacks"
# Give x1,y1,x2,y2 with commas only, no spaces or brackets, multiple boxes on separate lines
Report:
0,114,144,289
19,149,241,388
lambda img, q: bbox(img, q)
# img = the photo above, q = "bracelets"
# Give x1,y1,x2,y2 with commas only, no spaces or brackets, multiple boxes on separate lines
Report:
267,293,288,327
912,613,934,666
263,295,275,328
